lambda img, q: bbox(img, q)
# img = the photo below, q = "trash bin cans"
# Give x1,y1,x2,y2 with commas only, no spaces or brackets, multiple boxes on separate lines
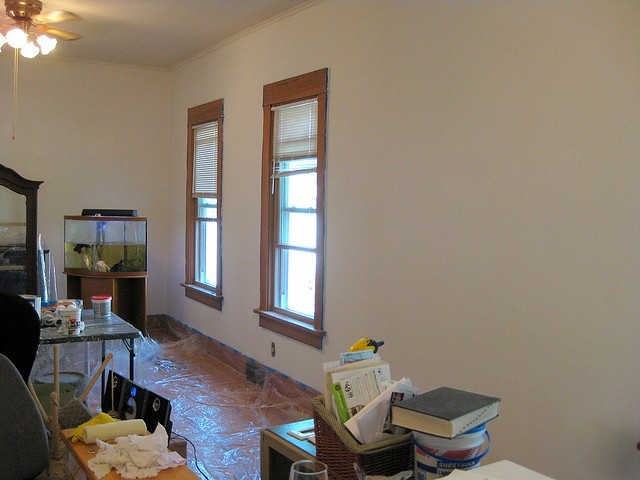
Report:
28,383,79,414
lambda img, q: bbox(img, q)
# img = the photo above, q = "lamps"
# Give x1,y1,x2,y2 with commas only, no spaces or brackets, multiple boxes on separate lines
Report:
0,19,60,140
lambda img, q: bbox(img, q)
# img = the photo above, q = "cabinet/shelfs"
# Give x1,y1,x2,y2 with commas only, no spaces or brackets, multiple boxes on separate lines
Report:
62,271,149,339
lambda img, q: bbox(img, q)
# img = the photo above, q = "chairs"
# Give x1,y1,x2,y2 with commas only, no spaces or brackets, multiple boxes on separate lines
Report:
1,291,41,385
0,353,49,480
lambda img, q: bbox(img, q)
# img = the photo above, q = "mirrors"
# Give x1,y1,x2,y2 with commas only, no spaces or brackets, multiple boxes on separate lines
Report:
0,163,44,296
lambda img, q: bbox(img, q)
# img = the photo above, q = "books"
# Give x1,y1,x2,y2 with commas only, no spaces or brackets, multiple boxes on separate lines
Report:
322,349,416,444
392,387,502,441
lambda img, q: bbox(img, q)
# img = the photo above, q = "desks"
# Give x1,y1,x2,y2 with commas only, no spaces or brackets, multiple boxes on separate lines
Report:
60,428,201,480
260,418,316,480
39,308,145,409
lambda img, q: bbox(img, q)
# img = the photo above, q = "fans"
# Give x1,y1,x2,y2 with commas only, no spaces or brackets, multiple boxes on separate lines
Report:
0,0,85,42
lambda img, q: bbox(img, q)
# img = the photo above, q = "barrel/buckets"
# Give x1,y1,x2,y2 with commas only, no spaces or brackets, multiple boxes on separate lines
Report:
412,423,490,480
60,308,82,335
32,382,78,417
35,370,90,414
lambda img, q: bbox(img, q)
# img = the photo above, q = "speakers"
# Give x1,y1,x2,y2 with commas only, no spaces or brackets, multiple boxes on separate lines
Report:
118,378,145,423
141,390,173,446
104,371,125,419
